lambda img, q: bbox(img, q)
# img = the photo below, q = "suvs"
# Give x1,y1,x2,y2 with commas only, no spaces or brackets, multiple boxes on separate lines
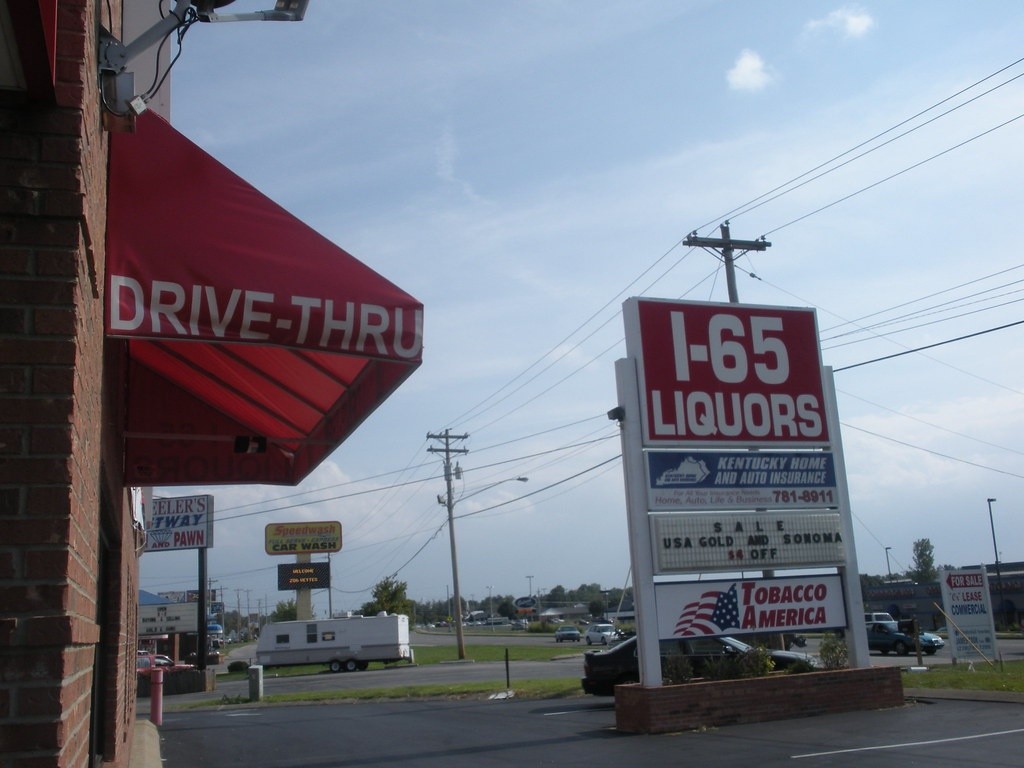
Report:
865,612,896,628
135,654,195,678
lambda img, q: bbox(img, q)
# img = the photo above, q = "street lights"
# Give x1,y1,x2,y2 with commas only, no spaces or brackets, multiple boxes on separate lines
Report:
885,546,895,602
525,575,534,596
444,476,529,660
987,498,1010,632
486,585,495,627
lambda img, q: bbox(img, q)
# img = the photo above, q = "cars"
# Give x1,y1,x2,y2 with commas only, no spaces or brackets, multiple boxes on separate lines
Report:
582,631,824,696
584,624,625,646
866,621,946,656
208,646,220,656
555,625,581,642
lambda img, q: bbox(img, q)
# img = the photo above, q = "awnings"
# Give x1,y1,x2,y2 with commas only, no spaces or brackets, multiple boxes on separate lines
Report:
107,96,424,486
